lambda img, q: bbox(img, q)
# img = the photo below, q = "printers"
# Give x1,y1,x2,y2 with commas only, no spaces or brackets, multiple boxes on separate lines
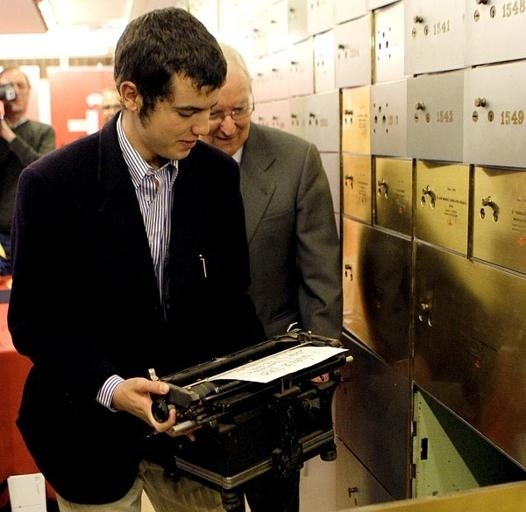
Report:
145,327,353,511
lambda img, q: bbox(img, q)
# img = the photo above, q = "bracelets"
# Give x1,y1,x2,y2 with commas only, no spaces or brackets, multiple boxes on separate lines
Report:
0,115,4,120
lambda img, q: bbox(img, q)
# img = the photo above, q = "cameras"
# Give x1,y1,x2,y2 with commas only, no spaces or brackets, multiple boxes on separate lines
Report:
0,83,17,102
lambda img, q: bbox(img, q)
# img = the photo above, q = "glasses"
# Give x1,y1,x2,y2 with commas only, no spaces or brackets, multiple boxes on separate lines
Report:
208,94,255,124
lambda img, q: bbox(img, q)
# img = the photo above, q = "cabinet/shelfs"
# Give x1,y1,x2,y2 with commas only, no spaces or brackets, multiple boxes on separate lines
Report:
218,1,525,512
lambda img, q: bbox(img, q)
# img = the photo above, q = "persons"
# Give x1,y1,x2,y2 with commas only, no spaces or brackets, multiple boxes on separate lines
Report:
200,43,343,512
101,87,122,125
0,65,56,347
3,5,332,511
